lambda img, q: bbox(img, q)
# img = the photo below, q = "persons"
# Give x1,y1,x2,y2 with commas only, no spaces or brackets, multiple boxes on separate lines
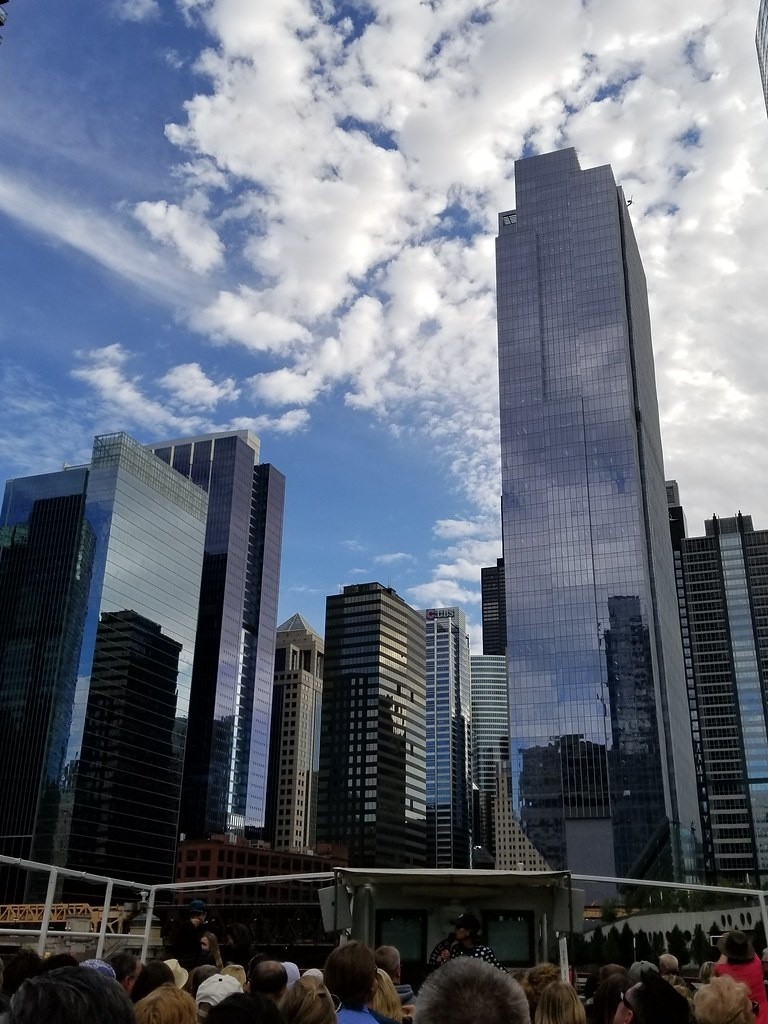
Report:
0,900,768,1024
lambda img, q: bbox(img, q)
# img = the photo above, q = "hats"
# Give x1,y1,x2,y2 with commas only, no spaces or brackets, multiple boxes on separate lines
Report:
188,899,207,912
78,960,116,979
163,959,189,990
628,960,659,980
449,912,480,934
717,929,754,961
195,974,245,1018
302,968,323,981
281,962,300,986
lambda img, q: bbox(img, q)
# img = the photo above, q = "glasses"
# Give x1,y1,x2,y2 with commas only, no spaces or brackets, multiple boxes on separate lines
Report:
613,990,638,1021
